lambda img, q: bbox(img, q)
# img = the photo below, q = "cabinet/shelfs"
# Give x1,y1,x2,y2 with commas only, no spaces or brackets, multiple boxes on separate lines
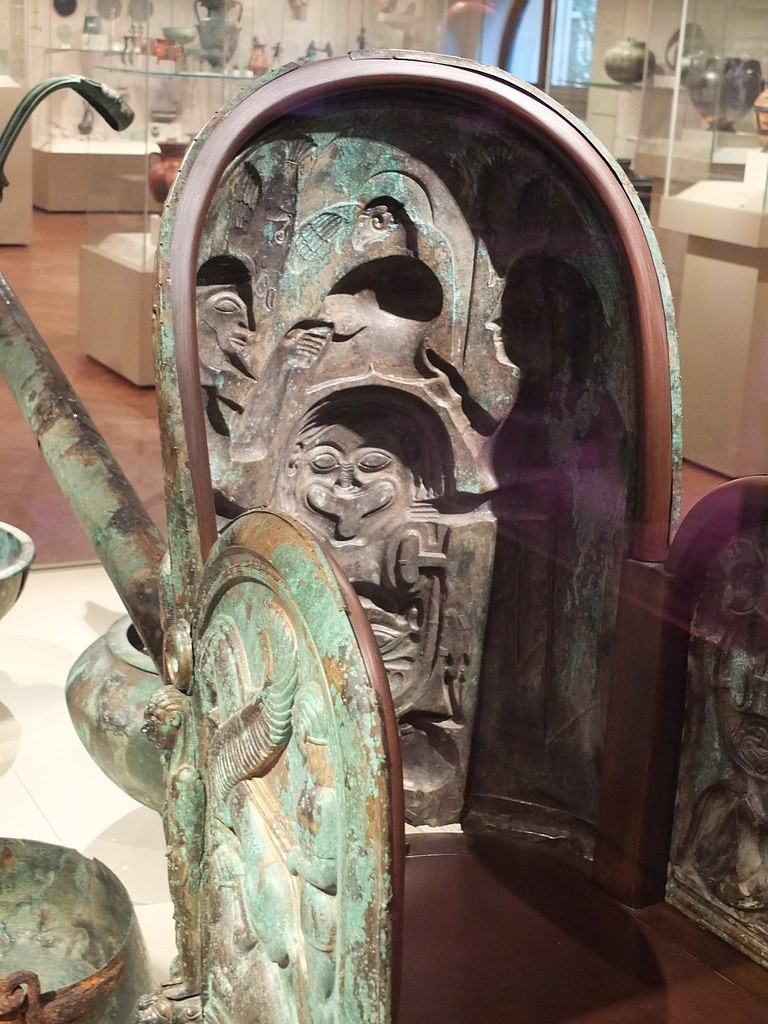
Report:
0,1,768,477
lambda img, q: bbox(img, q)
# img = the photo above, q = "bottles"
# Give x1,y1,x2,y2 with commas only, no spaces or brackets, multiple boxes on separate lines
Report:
604,41,655,84
249,44,269,77
193,0,243,73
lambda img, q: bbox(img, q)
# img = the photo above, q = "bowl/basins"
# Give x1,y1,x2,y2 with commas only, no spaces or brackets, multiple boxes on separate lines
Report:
1,835,135,1024
162,27,197,42
